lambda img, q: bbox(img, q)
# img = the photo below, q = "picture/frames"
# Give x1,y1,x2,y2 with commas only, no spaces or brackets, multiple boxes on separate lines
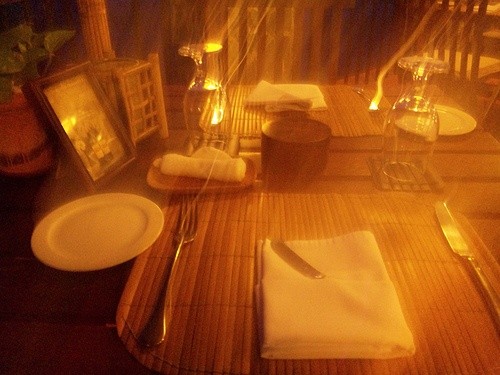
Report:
20,61,139,195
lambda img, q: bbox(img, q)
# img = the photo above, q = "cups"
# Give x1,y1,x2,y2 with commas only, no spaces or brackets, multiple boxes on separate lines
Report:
262,119,331,186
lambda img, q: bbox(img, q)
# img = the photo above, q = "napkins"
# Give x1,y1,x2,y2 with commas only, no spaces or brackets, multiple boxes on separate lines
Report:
244,79,328,113
160,147,247,183
254,232,417,361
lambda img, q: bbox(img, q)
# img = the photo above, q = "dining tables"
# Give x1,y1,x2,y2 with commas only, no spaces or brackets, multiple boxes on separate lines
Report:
37,77,500,375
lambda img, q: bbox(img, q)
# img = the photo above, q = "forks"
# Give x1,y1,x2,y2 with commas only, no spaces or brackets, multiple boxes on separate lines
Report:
357,88,386,120
141,196,198,347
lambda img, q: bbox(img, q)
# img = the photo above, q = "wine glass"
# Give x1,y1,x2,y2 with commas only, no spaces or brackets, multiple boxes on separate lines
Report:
178,43,231,136
380,56,449,181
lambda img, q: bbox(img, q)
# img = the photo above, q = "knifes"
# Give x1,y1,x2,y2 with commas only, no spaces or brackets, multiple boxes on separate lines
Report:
271,239,368,311
434,201,500,330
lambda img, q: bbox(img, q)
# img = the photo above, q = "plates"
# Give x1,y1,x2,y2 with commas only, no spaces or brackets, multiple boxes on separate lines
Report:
32,193,165,271
147,157,255,195
436,104,476,135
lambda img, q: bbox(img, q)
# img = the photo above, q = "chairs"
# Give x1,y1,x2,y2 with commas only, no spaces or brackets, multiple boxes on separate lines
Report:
399,0,500,130
